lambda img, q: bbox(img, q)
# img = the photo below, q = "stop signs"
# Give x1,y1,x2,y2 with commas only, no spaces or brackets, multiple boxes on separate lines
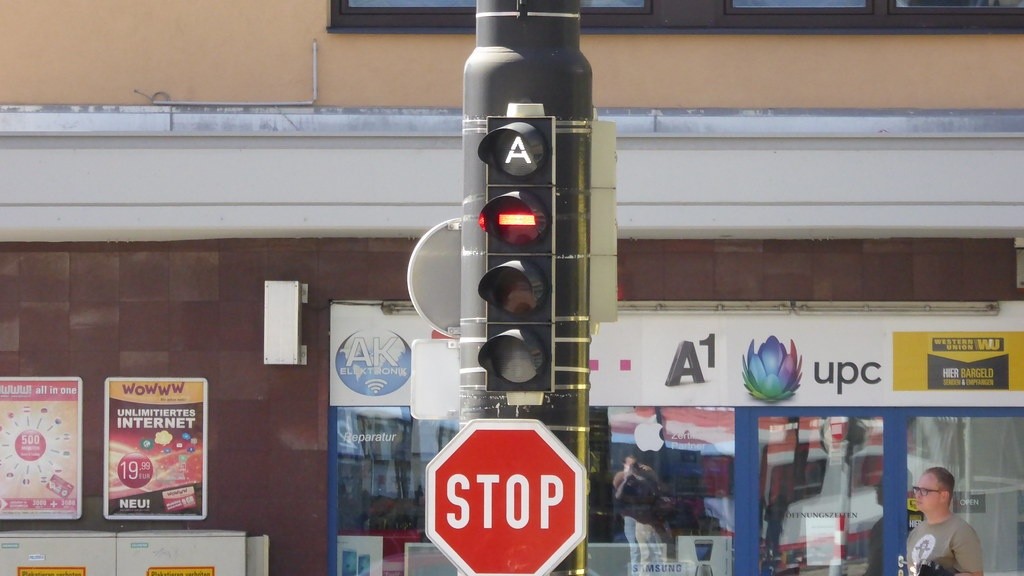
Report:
421,415,587,576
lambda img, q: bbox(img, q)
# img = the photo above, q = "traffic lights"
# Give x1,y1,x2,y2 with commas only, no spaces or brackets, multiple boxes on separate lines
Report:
476,113,558,396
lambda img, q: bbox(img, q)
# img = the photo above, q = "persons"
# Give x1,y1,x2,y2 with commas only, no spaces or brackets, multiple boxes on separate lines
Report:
612,452,665,576
906,466,984,576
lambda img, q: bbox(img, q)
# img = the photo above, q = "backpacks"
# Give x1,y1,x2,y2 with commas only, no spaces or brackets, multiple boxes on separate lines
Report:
615,471,645,504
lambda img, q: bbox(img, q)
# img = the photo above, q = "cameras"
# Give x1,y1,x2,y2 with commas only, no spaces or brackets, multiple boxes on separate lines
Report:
630,463,641,475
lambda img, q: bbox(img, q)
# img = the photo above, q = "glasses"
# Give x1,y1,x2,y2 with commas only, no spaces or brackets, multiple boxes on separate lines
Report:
873,484,883,490
911,486,942,497
